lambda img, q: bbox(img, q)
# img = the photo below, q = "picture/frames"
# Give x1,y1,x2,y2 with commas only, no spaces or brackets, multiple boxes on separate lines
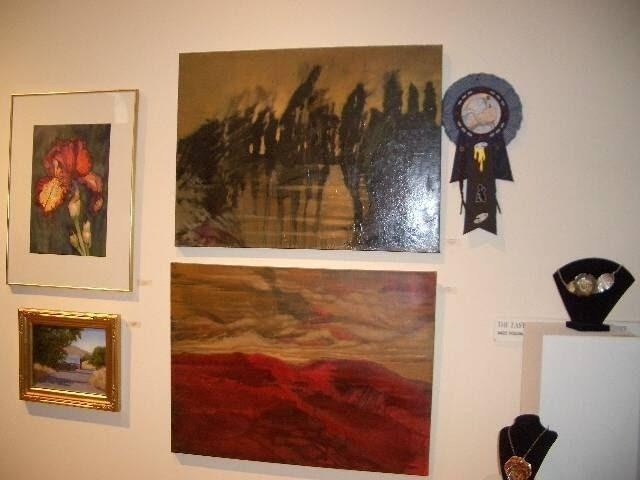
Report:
7,89,138,293
16,307,123,414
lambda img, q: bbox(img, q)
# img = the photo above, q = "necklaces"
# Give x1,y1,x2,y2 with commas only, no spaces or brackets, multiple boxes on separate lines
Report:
556,264,623,296
504,426,547,480
